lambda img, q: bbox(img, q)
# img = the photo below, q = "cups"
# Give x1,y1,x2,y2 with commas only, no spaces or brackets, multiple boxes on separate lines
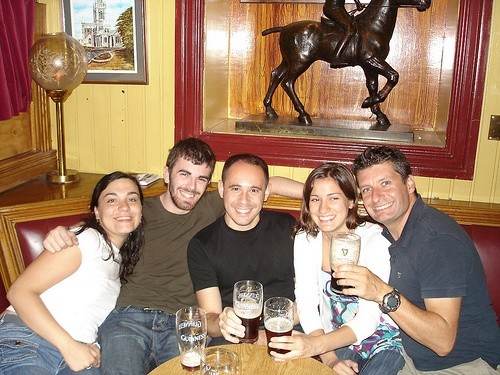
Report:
233,280,263,343
175,305,207,371
200,348,242,375
264,297,294,357
329,232,361,295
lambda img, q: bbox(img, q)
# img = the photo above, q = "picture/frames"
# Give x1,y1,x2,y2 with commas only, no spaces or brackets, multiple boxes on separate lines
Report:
59,0,149,85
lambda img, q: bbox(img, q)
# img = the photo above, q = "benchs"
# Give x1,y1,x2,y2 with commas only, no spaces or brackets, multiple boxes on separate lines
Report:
0,187,500,328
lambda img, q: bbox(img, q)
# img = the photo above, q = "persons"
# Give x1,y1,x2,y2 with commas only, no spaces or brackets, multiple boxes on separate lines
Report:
41,137,305,375
332,146,500,375
187,153,304,347
0,172,147,375
322,0,362,67
268,162,404,375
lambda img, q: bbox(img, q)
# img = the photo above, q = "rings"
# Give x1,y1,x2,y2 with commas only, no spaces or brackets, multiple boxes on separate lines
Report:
87,365,92,370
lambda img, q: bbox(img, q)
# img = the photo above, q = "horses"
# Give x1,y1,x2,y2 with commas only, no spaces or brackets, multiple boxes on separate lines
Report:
261,0,432,127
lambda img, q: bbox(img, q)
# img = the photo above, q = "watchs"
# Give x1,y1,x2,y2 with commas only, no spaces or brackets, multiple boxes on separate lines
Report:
379,288,401,315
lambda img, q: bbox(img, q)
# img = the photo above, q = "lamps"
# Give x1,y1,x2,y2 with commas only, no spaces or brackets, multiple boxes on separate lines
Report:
29,32,88,184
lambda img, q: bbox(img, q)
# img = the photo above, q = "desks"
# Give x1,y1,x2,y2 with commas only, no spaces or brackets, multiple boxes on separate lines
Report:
145,343,338,375
